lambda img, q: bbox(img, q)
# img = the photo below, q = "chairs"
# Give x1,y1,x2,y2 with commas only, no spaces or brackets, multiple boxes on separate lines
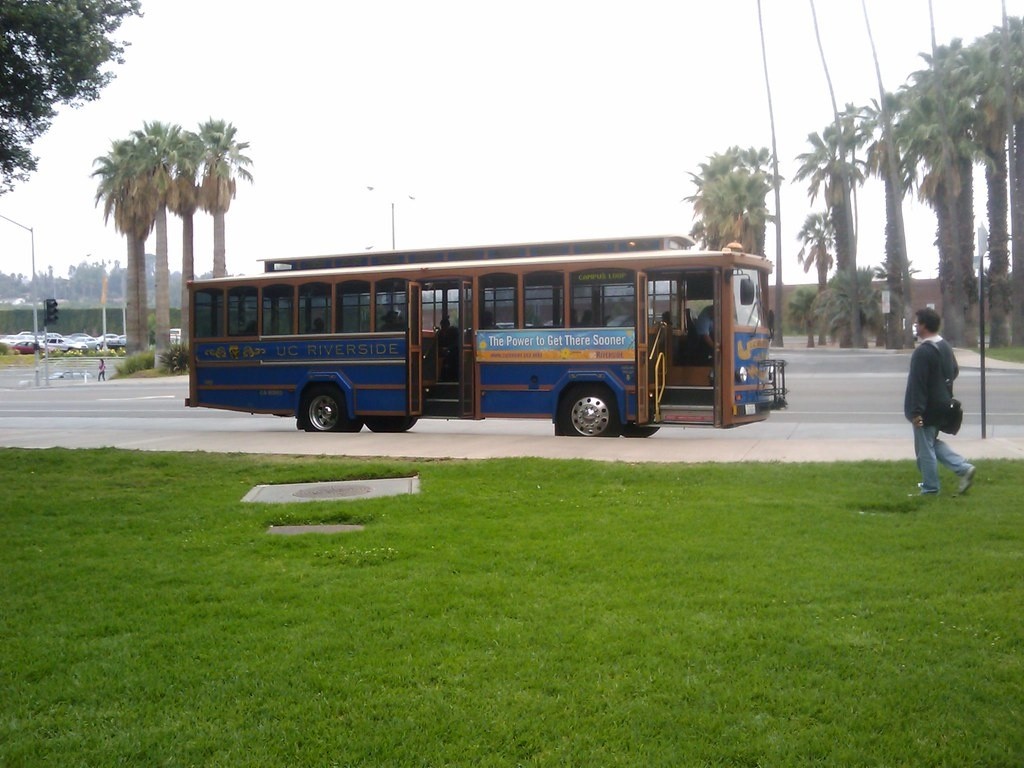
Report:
686,309,707,363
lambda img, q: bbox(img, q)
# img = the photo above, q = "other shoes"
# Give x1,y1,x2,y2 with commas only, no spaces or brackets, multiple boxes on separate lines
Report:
907,483,940,497
958,465,976,495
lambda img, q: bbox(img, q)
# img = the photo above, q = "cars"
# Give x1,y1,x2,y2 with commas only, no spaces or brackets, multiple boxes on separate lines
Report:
901,318,918,340
0,331,127,350
12,341,56,355
43,337,89,353
170,328,181,345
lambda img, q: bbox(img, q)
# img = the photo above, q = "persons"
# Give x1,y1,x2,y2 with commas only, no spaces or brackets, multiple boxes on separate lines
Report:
695,305,714,359
433,320,458,378
98,359,106,381
903,307,976,496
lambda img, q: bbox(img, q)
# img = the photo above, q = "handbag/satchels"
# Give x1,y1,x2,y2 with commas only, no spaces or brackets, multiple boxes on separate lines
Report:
939,399,962,435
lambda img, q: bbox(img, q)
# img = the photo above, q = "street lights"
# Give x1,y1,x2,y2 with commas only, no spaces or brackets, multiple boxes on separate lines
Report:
86,246,108,351
108,253,127,335
368,185,414,250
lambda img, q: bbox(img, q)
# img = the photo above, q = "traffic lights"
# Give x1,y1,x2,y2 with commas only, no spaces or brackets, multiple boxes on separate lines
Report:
45,299,58,325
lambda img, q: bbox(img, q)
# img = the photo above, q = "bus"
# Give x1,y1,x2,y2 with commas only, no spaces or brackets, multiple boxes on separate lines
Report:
185,236,790,437
